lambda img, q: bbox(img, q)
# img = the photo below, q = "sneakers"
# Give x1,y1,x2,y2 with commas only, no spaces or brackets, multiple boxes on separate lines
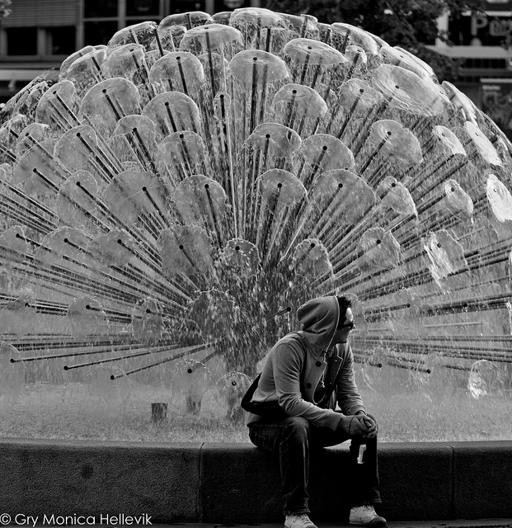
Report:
283,513,318,528
348,504,388,527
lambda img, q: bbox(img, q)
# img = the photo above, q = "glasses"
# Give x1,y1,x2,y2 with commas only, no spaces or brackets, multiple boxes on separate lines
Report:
340,320,355,332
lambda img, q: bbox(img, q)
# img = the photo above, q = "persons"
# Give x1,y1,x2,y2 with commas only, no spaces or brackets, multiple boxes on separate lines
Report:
241,295,389,528
